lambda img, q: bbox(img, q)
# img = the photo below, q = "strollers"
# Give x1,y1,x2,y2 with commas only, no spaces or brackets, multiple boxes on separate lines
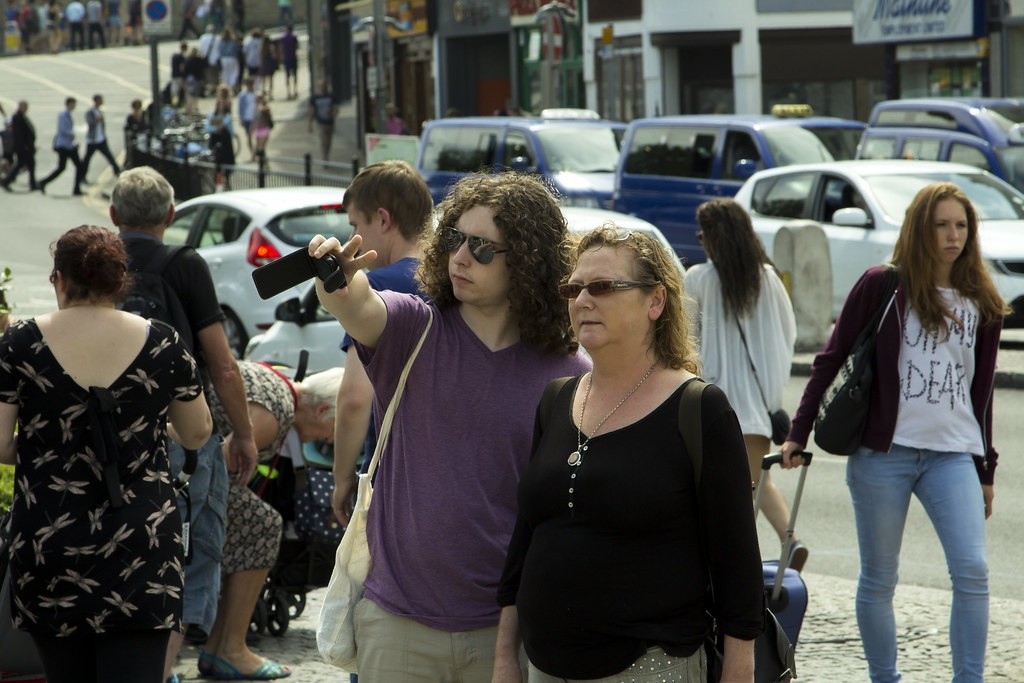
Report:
242,422,333,638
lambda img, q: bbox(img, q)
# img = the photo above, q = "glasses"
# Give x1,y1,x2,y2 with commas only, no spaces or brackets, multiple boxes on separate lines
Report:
697,231,706,243
558,279,657,299
440,227,512,264
49,272,68,283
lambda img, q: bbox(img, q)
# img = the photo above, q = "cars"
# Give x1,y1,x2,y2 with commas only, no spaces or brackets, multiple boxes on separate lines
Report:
242,96,1024,379
158,182,369,363
731,157,1024,358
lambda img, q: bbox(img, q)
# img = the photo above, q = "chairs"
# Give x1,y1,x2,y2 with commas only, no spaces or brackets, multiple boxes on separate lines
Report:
842,185,858,210
222,216,248,243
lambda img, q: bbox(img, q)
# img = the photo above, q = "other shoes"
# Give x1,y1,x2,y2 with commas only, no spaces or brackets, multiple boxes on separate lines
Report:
786,538,807,572
197,653,290,680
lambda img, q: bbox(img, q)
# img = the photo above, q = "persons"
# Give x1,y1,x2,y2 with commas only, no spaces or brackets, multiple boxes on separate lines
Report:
2,78,275,195
309,171,595,682
775,181,1004,682
380,102,532,136
0,224,213,683
307,79,342,172
679,197,809,572
0,0,294,55
92,161,433,681
169,22,303,102
493,226,765,683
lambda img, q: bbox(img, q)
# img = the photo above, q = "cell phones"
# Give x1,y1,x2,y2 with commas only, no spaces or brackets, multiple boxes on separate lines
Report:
307,249,344,290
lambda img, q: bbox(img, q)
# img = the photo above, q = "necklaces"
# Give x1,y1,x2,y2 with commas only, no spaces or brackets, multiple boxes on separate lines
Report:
567,359,662,465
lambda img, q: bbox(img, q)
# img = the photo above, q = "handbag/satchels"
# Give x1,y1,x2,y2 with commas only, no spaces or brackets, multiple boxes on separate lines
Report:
316,473,378,670
705,605,796,683
814,265,899,456
768,410,791,444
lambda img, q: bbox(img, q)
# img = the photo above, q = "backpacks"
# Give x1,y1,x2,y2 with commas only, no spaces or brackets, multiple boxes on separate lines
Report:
116,244,193,351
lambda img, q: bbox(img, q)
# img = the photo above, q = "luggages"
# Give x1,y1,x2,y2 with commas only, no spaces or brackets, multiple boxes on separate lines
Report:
753,450,815,683
